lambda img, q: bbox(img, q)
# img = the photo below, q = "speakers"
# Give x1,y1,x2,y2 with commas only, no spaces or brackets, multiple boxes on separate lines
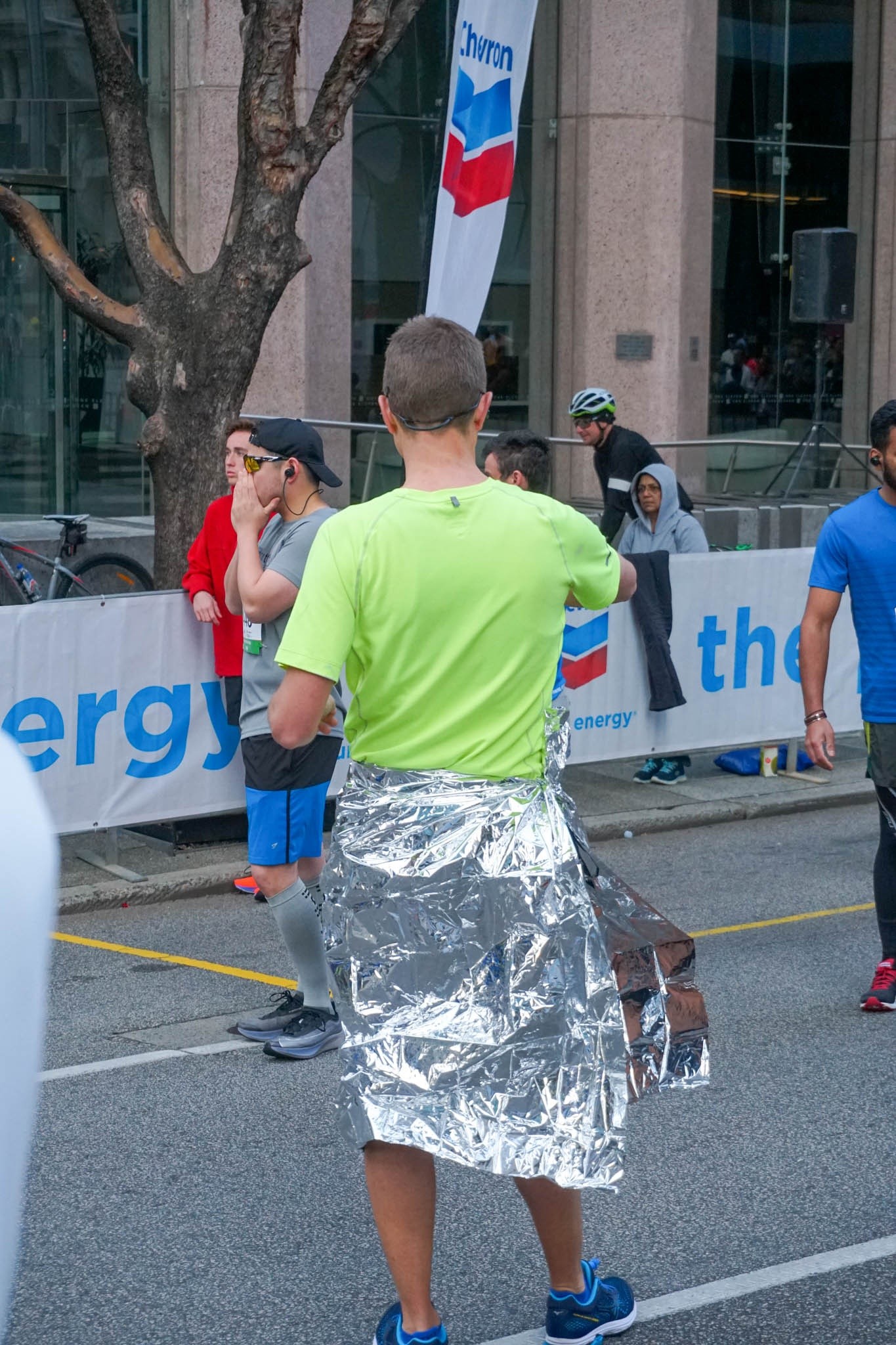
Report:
790,226,858,322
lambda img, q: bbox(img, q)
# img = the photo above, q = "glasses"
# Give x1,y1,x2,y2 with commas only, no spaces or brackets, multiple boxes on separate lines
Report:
573,412,608,429
243,453,290,473
636,483,660,494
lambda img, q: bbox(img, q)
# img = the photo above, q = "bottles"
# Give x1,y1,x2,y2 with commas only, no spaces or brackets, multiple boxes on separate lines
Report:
16,562,42,601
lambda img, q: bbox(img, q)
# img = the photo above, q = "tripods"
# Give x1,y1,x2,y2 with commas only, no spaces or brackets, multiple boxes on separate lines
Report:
763,324,879,501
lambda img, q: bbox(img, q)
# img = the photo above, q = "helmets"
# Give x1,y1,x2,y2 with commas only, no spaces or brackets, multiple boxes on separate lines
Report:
568,388,616,417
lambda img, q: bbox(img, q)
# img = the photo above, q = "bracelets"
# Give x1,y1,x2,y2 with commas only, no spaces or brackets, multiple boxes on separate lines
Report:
804,709,827,726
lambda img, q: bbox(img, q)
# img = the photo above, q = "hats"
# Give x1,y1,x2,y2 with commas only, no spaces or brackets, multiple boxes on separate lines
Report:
249,417,343,488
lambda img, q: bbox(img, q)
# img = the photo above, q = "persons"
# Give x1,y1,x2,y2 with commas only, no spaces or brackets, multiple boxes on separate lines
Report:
224,417,347,1059
351,320,521,425
480,427,566,701
799,399,896,1014
617,464,709,785
267,314,638,1345
709,335,844,430
569,386,697,547
181,419,279,902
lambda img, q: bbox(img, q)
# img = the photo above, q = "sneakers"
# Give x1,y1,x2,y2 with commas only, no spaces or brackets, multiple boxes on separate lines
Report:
545,1258,637,1345
859,957,896,1011
263,1009,345,1059
236,990,336,1042
373,1300,449,1345
233,865,259,894
254,888,267,900
633,758,663,784
651,759,687,785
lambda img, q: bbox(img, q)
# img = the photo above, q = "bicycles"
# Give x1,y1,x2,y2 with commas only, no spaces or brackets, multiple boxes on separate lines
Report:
1,513,154,605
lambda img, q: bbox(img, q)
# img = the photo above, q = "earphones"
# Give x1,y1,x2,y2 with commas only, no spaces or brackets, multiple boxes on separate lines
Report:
871,456,881,466
285,468,294,476
